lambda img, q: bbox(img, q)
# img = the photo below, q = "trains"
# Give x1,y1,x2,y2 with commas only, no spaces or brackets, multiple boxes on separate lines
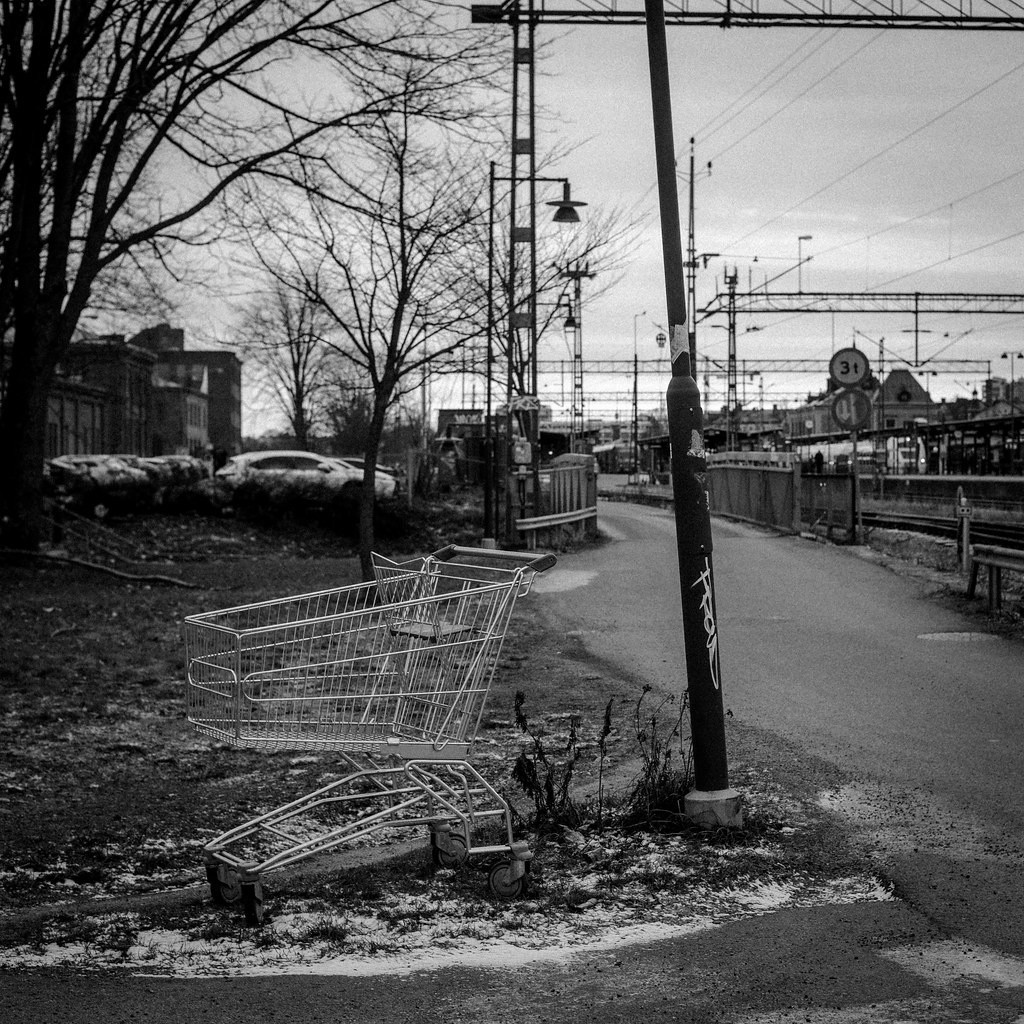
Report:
594,445,640,475
795,434,927,476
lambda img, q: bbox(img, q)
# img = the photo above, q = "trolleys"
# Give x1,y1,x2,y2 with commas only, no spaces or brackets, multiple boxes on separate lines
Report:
187,542,558,926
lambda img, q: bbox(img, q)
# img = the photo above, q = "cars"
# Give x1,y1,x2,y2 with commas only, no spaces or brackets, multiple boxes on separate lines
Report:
330,457,397,479
53,451,209,486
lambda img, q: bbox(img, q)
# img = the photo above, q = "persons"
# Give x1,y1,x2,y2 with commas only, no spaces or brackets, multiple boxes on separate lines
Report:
929,447,939,475
810,455,816,472
816,450,824,473
948,446,976,475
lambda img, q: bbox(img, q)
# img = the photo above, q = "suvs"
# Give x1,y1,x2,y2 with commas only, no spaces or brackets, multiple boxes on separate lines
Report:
215,450,384,498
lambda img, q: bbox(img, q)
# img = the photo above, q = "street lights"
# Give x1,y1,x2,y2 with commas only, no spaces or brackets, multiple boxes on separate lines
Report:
526,301,582,521
919,371,938,424
483,175,590,535
1002,349,1024,415
